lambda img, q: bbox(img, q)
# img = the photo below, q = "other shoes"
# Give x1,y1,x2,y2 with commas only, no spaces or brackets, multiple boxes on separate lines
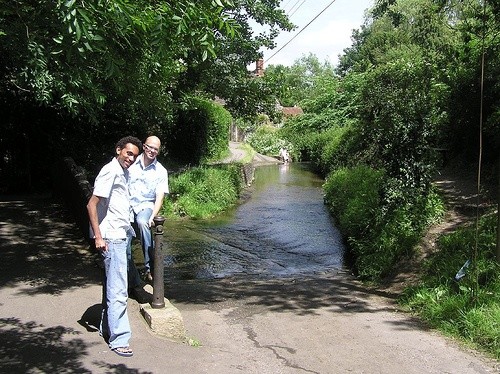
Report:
145,273,153,282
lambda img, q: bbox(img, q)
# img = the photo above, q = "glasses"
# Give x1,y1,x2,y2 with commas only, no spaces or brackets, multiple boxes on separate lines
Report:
146,144,160,153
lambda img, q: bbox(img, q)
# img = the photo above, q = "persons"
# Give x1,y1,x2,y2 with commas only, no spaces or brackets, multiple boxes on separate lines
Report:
279,164,289,175
86,136,143,356
279,147,288,163
127,135,170,288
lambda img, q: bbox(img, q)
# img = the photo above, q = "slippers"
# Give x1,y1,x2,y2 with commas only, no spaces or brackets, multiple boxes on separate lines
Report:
114,346,133,356
